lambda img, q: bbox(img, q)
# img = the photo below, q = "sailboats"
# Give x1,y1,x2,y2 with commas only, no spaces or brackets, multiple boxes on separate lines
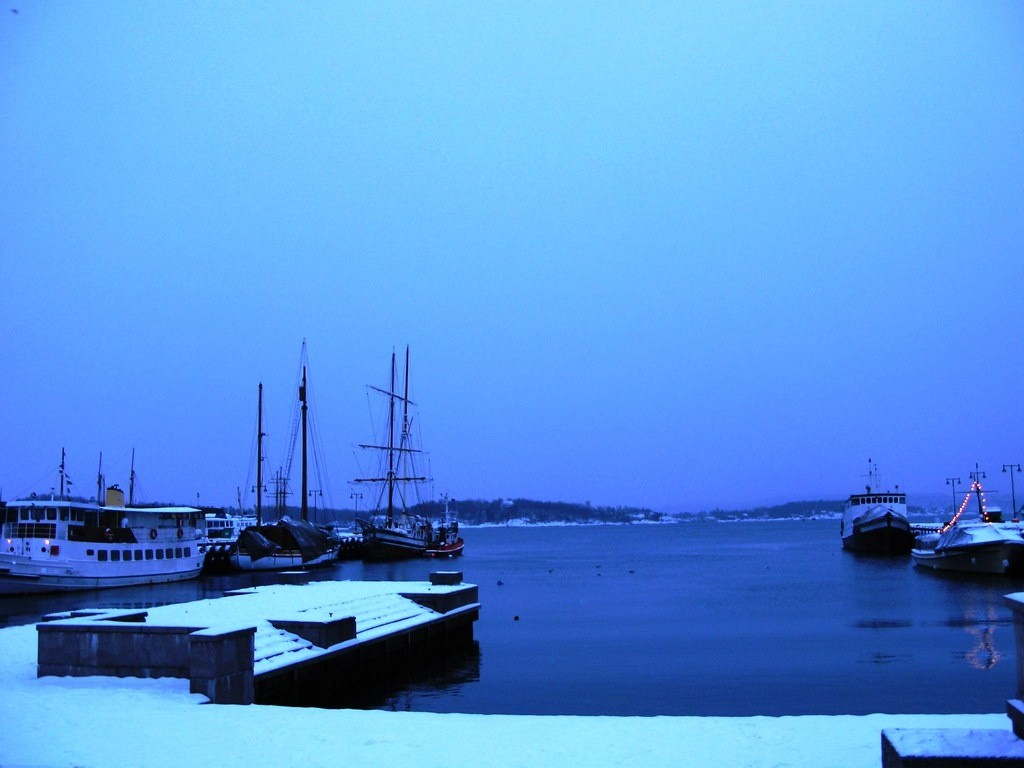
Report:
228,335,345,572
345,341,464,559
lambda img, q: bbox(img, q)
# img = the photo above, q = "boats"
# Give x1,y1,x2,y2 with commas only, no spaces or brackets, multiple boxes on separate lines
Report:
0,447,210,592
207,512,260,544
423,491,466,559
841,457,912,548
910,465,1024,572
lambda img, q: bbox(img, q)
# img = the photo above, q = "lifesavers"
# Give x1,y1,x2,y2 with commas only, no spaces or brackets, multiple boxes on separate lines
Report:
177,528,184,539
149,528,158,540
